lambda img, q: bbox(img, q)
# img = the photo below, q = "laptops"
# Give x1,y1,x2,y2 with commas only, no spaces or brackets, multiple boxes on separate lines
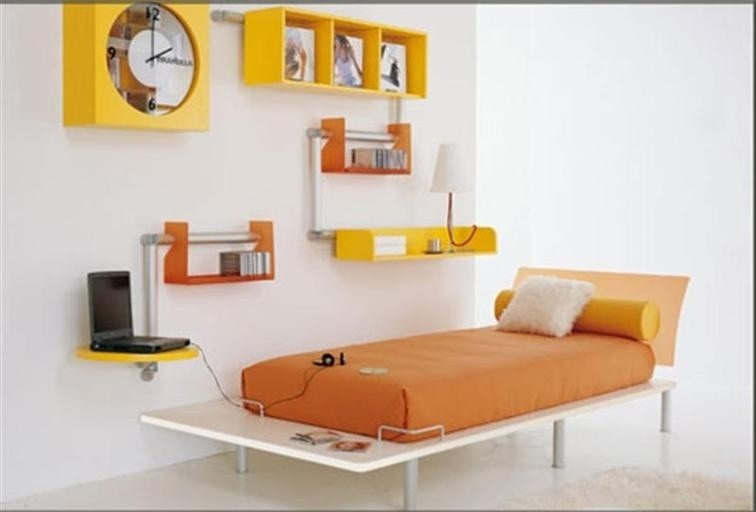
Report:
86,270,189,354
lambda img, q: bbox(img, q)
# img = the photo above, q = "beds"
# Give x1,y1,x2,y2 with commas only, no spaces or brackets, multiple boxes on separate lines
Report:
138,265,695,509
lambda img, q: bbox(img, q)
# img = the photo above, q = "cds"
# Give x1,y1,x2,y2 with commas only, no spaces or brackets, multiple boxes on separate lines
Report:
359,367,390,375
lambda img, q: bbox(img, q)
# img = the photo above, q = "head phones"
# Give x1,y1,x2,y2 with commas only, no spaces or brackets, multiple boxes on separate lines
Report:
313,350,347,365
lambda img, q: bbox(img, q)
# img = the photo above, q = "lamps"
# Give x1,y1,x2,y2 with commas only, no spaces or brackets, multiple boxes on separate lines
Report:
430,142,477,252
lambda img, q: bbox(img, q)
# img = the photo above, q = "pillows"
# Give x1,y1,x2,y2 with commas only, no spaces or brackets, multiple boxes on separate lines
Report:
493,274,661,344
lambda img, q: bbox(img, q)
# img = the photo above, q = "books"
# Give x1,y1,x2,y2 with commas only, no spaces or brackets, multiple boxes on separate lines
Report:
288,430,372,454
352,146,408,171
219,251,271,277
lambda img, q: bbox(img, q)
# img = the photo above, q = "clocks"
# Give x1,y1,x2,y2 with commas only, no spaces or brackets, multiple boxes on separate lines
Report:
62,1,211,133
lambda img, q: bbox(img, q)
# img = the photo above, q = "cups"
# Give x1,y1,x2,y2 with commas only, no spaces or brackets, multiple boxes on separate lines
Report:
427,239,440,251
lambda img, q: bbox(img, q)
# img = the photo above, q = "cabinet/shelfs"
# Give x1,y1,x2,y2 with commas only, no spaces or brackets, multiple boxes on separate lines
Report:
243,7,429,101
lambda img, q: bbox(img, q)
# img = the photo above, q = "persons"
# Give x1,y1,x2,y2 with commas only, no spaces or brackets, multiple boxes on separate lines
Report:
283,26,307,81
389,58,401,88
332,33,362,86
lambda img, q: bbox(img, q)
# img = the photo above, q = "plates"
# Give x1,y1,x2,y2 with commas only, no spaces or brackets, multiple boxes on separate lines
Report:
423,250,445,254
